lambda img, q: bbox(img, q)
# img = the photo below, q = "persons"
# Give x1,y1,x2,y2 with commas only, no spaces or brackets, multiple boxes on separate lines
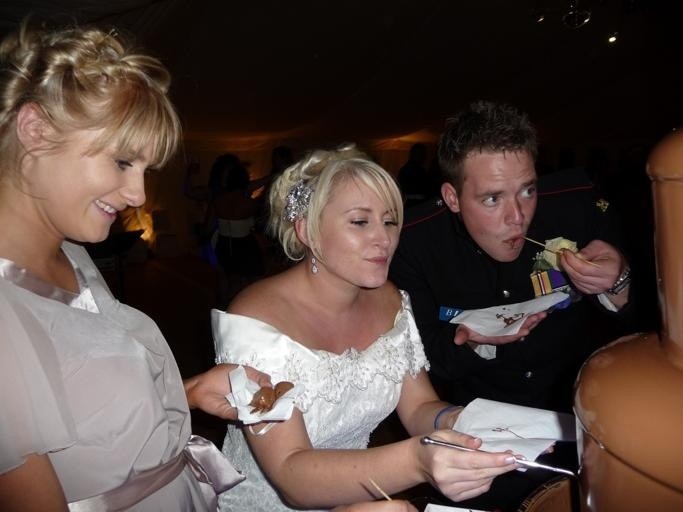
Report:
0,24,304,511
387,116,644,511
209,144,558,511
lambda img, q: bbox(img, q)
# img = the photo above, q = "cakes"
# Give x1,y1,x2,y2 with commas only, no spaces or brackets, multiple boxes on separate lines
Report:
250,381,292,410
572,127,683,512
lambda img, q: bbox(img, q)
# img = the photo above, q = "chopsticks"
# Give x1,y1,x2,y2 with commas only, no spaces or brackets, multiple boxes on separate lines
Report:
419,434,576,478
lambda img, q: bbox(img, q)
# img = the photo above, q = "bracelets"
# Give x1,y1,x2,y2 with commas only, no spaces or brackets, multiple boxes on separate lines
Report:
605,267,631,297
432,402,455,431
